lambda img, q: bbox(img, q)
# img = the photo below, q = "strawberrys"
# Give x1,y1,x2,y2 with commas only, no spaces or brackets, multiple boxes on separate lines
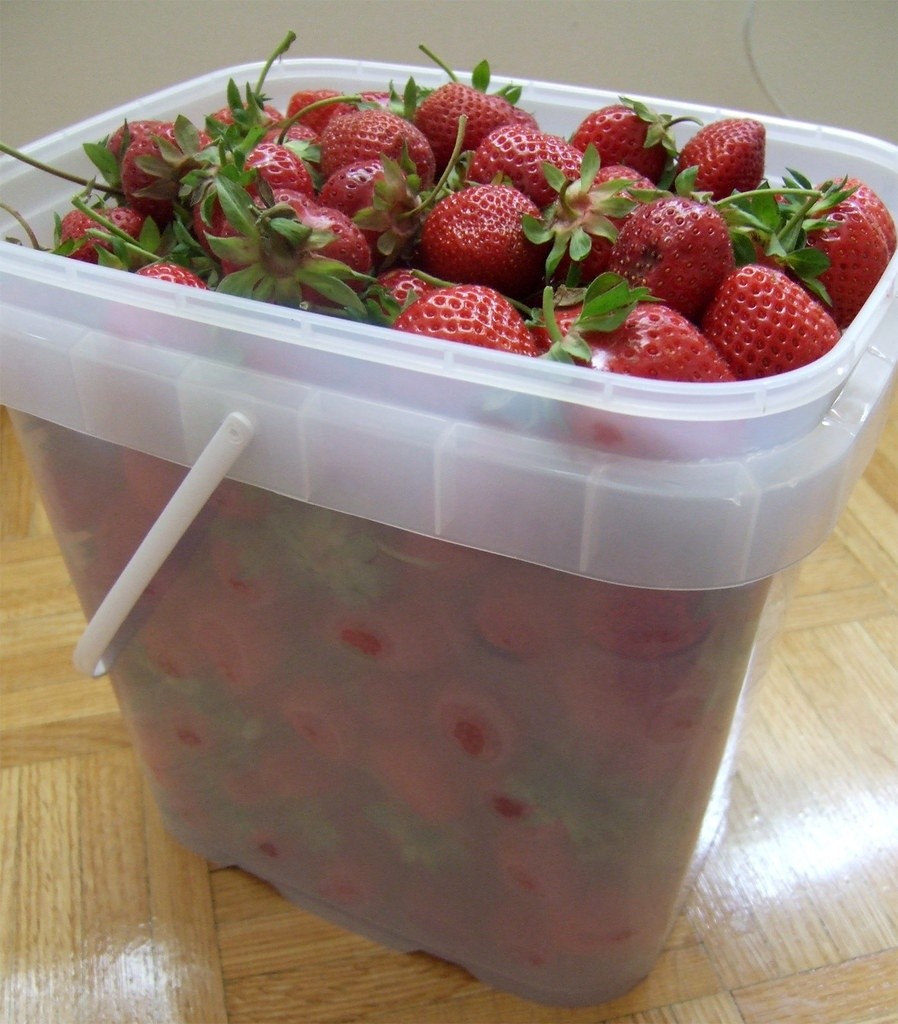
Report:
14,79,898,1005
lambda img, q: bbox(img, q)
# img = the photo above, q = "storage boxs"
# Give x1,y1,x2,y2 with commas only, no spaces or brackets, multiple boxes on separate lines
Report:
0,58,898,1007
